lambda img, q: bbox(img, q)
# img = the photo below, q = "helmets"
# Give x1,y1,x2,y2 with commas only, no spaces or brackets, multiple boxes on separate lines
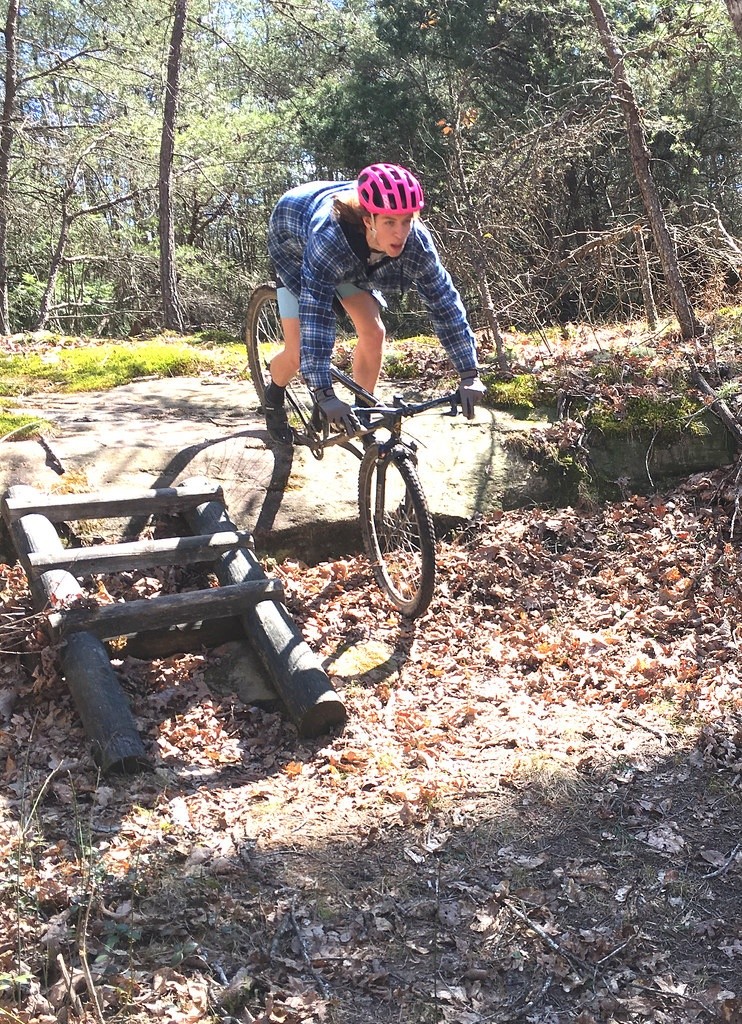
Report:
358,163,424,214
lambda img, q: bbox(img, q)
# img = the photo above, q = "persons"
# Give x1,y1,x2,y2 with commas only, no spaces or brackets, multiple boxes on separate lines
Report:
261,163,486,445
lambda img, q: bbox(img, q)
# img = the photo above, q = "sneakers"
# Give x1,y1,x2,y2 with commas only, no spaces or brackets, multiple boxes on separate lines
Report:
263,385,295,446
354,412,377,445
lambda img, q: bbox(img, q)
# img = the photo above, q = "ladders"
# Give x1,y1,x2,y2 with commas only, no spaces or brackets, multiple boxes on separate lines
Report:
0,476,354,777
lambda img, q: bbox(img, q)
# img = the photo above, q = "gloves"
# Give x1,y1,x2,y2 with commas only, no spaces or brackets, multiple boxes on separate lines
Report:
456,370,487,419
314,386,361,439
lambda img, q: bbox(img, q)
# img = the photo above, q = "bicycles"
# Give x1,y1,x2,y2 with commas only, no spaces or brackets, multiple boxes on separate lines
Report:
245,280,472,617
